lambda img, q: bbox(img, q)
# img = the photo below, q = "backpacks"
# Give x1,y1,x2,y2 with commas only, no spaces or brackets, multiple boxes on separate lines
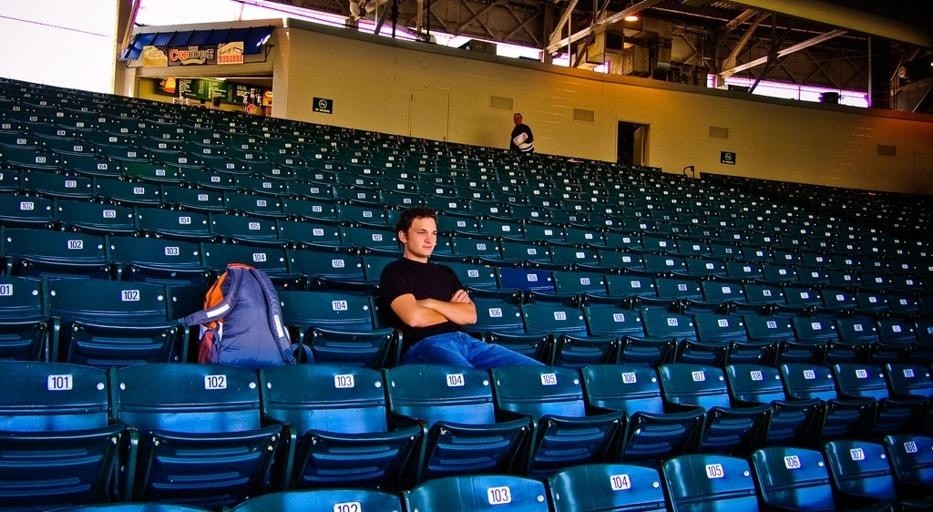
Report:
177,261,299,366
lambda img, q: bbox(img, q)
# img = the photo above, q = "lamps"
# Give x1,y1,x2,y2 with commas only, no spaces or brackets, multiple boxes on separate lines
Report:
625,2,639,22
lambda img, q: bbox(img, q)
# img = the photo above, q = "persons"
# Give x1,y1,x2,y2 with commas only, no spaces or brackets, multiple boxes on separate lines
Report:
197,99,209,109
209,97,221,110
510,112,533,153
376,205,547,368
